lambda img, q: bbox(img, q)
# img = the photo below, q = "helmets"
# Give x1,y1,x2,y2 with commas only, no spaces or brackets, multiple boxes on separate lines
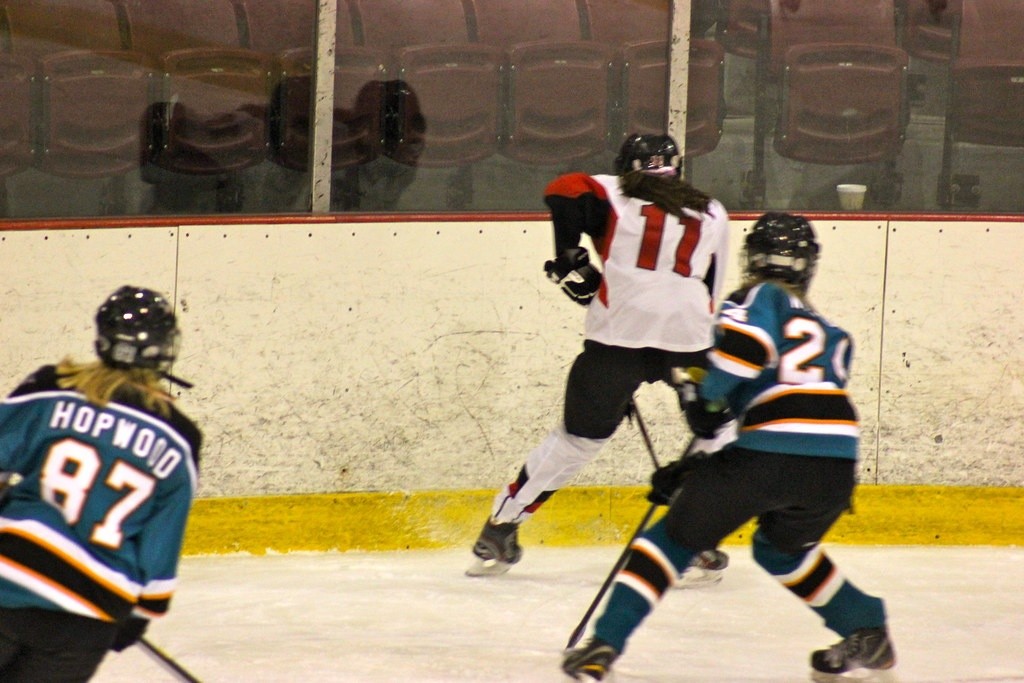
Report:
743,211,820,283
93,285,181,372
614,132,684,179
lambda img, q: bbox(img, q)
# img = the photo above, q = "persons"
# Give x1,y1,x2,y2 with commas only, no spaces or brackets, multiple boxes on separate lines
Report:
558,210,899,683
463,128,732,590
0,285,204,683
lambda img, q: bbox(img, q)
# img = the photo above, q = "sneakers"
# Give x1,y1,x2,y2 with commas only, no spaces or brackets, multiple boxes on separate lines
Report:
464,513,523,576
560,635,622,683
807,626,900,683
671,547,731,588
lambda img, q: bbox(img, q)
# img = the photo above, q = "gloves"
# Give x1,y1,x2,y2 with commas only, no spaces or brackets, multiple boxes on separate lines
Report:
683,398,736,440
544,246,602,306
108,613,150,653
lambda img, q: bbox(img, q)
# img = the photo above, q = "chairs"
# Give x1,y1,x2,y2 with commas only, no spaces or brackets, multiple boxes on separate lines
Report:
0,0,1024,211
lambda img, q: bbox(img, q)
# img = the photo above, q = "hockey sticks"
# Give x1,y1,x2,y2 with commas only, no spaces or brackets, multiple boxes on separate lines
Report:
629,403,732,570
565,427,706,653
131,637,205,683
